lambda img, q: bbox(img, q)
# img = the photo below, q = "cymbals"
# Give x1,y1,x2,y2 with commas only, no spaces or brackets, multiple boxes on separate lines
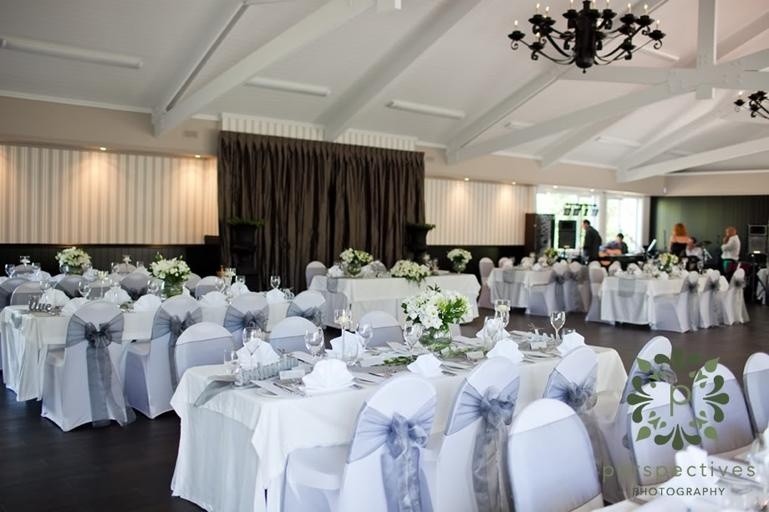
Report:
696,241,712,249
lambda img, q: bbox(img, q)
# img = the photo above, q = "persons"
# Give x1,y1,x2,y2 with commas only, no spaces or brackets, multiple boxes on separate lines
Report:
686,237,703,258
582,219,601,265
719,227,741,283
669,223,688,268
606,234,628,271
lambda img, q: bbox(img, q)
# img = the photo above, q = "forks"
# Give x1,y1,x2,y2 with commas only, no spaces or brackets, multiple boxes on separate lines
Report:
223,331,562,395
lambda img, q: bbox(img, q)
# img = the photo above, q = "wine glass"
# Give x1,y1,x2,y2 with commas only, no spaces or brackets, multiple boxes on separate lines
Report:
5,255,246,306
333,309,353,358
271,275,280,289
549,308,566,353
494,299,513,341
242,327,263,386
304,326,324,374
402,324,423,365
356,324,374,355
334,259,440,281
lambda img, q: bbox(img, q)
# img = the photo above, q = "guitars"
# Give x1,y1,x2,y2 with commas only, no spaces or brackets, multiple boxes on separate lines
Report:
600,247,622,265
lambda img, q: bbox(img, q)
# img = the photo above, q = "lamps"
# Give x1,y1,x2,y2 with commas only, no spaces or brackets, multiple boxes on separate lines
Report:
733,90,769,119
507,0,666,74
